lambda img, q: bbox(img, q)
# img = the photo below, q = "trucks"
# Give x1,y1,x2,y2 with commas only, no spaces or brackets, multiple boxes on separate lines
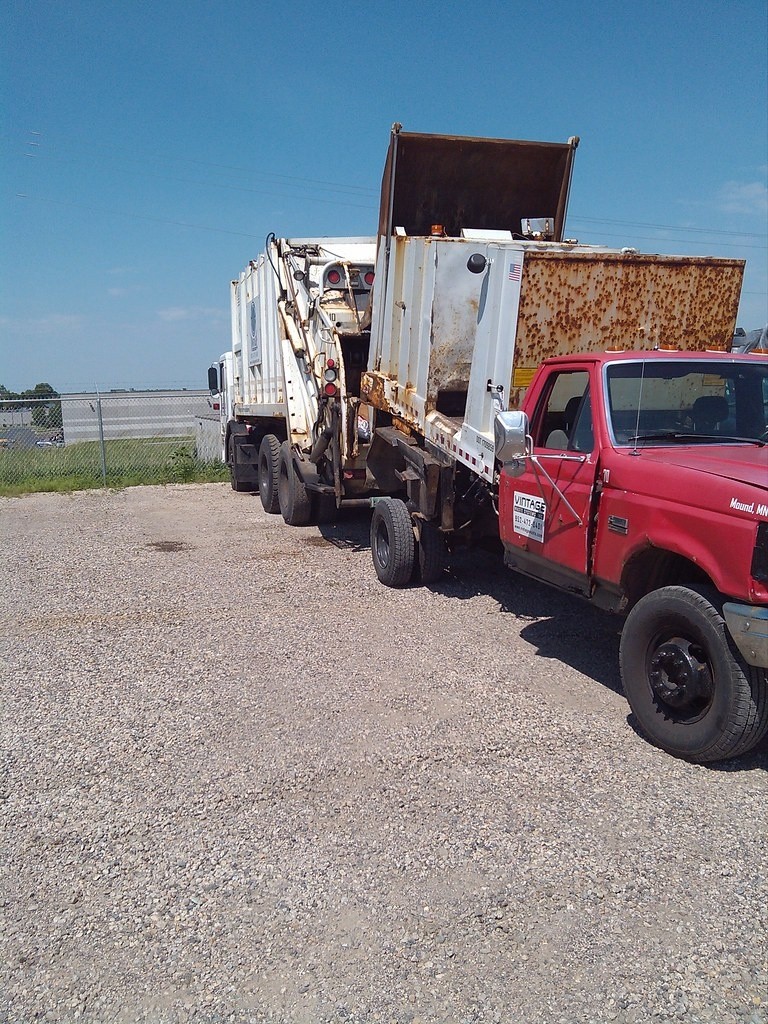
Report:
357,221,767,764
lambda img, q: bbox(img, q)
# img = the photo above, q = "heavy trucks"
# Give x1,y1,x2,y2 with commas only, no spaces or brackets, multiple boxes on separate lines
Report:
208,232,377,524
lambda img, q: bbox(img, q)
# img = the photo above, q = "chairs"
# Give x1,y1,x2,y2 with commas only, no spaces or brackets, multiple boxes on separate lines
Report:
690,396,729,434
565,396,593,449
